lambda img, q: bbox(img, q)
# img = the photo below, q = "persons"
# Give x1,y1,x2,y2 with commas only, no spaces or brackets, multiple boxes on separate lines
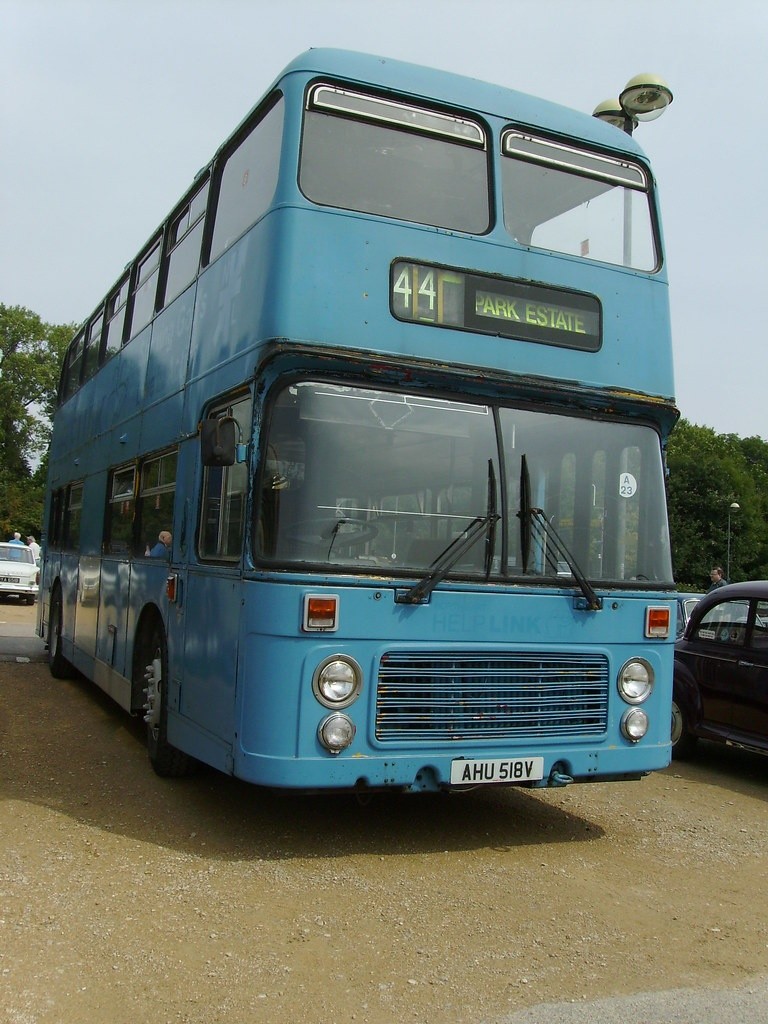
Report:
707,566,726,593
145,544,150,556
150,530,172,561
26,535,40,548
9,532,24,545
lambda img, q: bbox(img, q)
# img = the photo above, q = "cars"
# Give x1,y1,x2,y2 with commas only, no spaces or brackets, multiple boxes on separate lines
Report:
0,542,40,606
674,592,767,644
673,579,768,758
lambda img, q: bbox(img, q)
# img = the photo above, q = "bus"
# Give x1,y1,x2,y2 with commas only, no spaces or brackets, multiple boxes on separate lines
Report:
35,45,682,791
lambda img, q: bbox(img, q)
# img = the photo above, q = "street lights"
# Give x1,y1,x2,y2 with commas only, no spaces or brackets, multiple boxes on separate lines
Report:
587,73,674,271
727,502,741,584
